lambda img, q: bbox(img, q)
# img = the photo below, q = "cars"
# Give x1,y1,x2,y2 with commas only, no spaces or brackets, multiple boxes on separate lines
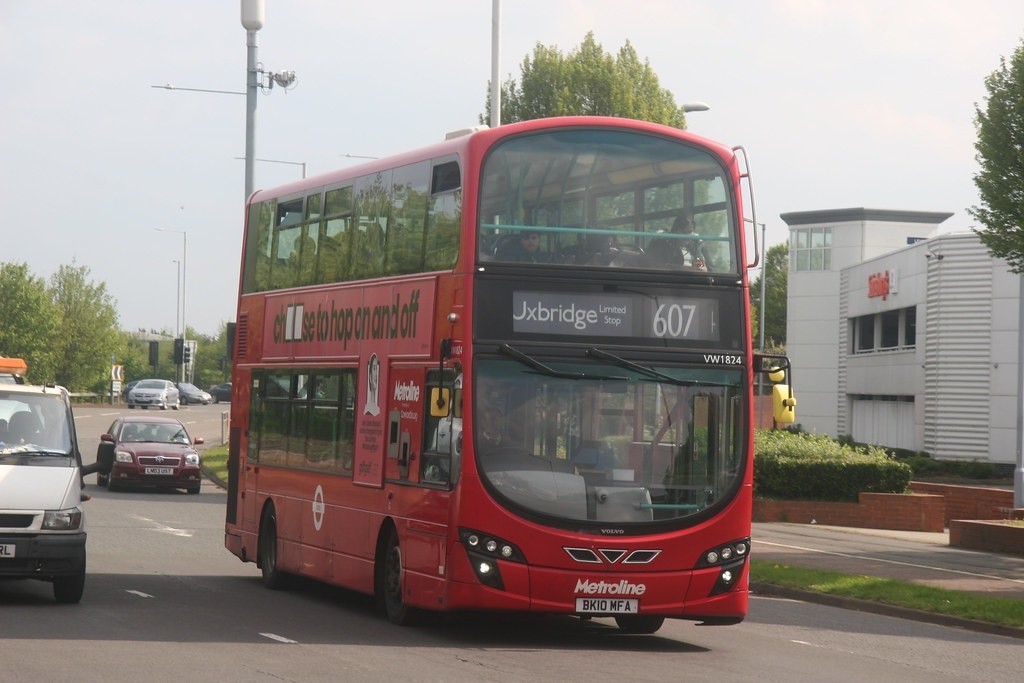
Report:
207,381,233,404
175,382,213,405
127,378,181,411
96,416,206,495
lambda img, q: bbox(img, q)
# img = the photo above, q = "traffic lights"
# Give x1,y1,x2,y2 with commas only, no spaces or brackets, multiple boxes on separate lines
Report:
183,345,191,365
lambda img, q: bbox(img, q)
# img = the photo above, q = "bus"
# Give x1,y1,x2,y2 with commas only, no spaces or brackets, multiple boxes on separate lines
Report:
224,116,797,636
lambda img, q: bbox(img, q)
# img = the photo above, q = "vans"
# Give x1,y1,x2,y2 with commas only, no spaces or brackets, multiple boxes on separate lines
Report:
0,358,87,604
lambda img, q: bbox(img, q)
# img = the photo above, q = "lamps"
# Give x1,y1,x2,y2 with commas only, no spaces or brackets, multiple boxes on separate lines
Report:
924,249,945,260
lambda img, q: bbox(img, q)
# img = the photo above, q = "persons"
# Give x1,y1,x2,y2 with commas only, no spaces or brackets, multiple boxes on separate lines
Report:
475,408,521,453
288,210,712,282
661,438,711,504
368,353,378,404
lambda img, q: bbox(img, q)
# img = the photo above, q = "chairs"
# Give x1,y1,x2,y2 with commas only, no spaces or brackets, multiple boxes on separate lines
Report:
641,229,668,269
8,411,40,445
489,233,520,256
121,425,138,442
157,427,170,441
